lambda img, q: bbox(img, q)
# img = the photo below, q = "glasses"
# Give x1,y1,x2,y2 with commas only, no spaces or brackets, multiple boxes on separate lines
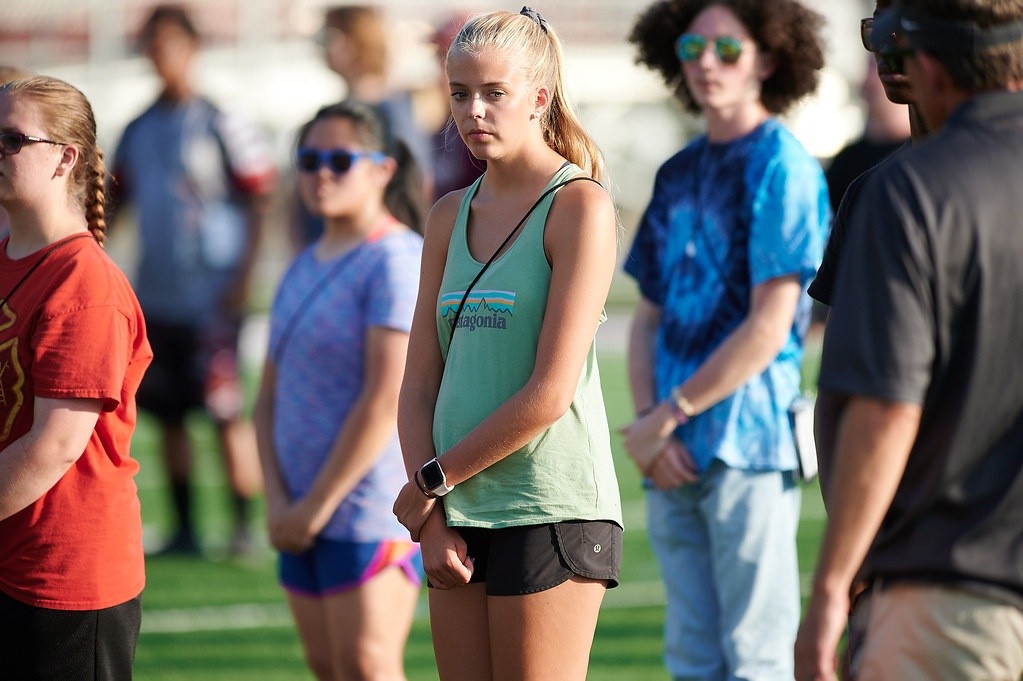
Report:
860,17,914,54
296,146,385,176
674,34,760,66
0,131,67,156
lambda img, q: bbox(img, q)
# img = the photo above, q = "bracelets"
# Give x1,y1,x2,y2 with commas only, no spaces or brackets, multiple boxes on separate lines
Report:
414,471,438,499
666,400,689,422
672,391,695,415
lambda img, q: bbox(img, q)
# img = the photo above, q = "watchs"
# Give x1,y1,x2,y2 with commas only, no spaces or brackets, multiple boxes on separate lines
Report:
420,457,454,496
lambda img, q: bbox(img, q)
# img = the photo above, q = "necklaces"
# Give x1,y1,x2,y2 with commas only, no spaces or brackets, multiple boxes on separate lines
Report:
0,236,94,310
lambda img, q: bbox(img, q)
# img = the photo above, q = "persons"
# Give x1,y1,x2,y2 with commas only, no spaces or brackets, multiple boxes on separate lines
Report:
619,0,832,681
393,6,623,681
255,101,425,681
794,0,1023,680
0,76,153,681
294,7,488,235
102,6,278,565
